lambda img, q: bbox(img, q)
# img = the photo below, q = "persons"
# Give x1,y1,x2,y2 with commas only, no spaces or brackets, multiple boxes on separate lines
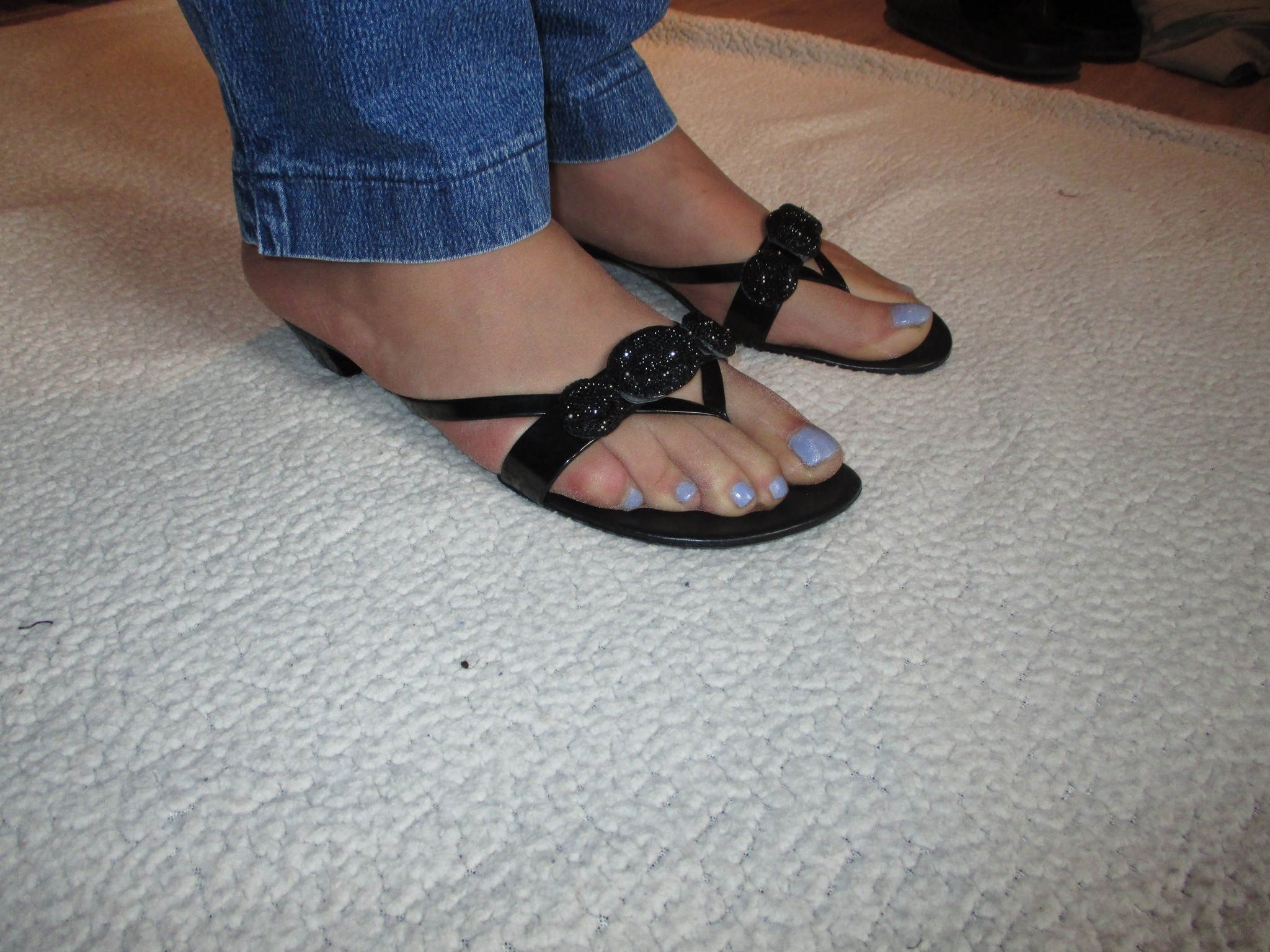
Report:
1140,0,1270,88
177,0,952,550
884,0,1139,84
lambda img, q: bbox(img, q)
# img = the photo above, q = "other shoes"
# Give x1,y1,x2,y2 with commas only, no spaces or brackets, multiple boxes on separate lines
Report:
1061,0,1143,64
881,0,1083,85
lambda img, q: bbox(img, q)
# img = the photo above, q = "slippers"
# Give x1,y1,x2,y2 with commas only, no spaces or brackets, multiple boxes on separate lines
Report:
283,309,863,551
577,204,951,375
